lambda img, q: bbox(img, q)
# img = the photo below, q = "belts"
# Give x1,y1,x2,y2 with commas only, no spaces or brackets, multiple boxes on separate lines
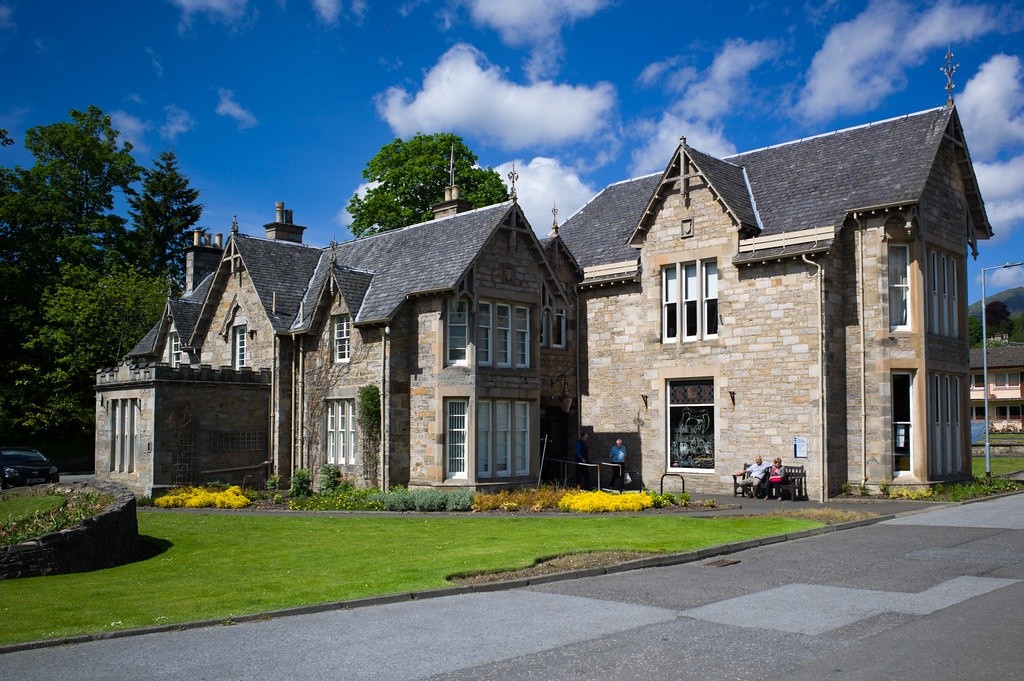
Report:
755,477,762,481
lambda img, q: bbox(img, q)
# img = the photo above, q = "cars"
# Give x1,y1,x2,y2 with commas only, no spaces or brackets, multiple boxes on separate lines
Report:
0,446,60,490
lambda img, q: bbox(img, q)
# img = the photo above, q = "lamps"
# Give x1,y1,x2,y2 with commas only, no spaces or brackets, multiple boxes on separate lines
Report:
551,374,573,413
248,326,257,340
222,331,228,344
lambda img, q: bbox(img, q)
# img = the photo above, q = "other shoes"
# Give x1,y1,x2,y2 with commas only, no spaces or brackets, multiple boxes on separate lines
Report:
763,495,770,501
746,492,753,499
776,497,782,501
583,485,592,491
753,494,758,499
607,486,613,489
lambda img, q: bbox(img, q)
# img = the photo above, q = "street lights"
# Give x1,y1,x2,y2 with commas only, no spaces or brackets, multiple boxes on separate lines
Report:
982,261,1023,478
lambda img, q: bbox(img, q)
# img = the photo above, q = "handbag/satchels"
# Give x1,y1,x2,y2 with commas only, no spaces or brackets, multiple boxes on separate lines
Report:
623,472,632,486
770,478,783,483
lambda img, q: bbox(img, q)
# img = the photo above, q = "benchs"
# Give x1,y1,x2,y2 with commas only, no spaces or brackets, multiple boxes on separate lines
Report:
732,463,804,501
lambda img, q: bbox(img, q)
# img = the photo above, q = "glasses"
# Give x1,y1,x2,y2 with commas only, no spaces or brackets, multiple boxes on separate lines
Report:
776,462,780,463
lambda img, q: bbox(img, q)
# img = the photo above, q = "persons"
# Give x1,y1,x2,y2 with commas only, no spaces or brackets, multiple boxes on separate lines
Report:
763,458,787,501
575,432,592,491
608,439,628,489
732,455,775,499
